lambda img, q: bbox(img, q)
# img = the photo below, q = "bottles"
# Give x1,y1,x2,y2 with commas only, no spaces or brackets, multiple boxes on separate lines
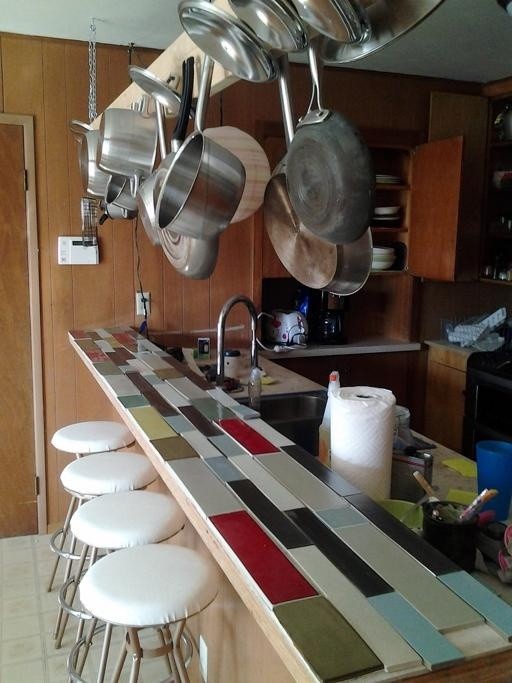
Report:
247,367,262,412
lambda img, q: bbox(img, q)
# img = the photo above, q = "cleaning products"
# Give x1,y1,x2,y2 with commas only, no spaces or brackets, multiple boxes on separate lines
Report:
319,371,341,468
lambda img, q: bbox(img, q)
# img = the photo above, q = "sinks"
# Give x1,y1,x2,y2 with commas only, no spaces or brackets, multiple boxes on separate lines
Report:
233,389,329,419
265,419,323,457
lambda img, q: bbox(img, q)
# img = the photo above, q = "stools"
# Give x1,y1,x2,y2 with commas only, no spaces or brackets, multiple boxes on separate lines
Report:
63,489,181,682
47,416,136,590
75,539,222,682
51,451,157,649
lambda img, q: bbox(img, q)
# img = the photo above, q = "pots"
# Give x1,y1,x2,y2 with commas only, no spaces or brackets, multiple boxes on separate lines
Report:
287,37,374,244
79,63,159,225
136,54,218,280
260,53,374,297
156,53,246,240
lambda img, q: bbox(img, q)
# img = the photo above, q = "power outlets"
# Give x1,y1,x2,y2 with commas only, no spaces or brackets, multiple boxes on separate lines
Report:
136,292,151,317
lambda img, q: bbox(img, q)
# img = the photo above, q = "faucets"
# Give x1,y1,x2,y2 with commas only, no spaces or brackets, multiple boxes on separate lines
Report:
216,294,258,388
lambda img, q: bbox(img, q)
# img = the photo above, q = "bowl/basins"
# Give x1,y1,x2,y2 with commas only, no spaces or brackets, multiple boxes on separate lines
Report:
371,246,396,270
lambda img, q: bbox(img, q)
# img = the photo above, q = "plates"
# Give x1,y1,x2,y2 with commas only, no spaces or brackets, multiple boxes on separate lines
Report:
371,205,402,222
374,172,401,185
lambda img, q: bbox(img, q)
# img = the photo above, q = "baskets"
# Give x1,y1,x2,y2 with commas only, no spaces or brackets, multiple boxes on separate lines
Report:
440,313,505,353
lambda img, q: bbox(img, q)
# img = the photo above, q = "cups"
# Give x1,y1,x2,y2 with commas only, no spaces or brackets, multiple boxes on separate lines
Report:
474,440,512,523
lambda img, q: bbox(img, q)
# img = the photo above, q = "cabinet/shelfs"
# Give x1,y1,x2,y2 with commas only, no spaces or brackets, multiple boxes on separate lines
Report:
426,74,512,286
423,347,468,454
217,117,465,351
